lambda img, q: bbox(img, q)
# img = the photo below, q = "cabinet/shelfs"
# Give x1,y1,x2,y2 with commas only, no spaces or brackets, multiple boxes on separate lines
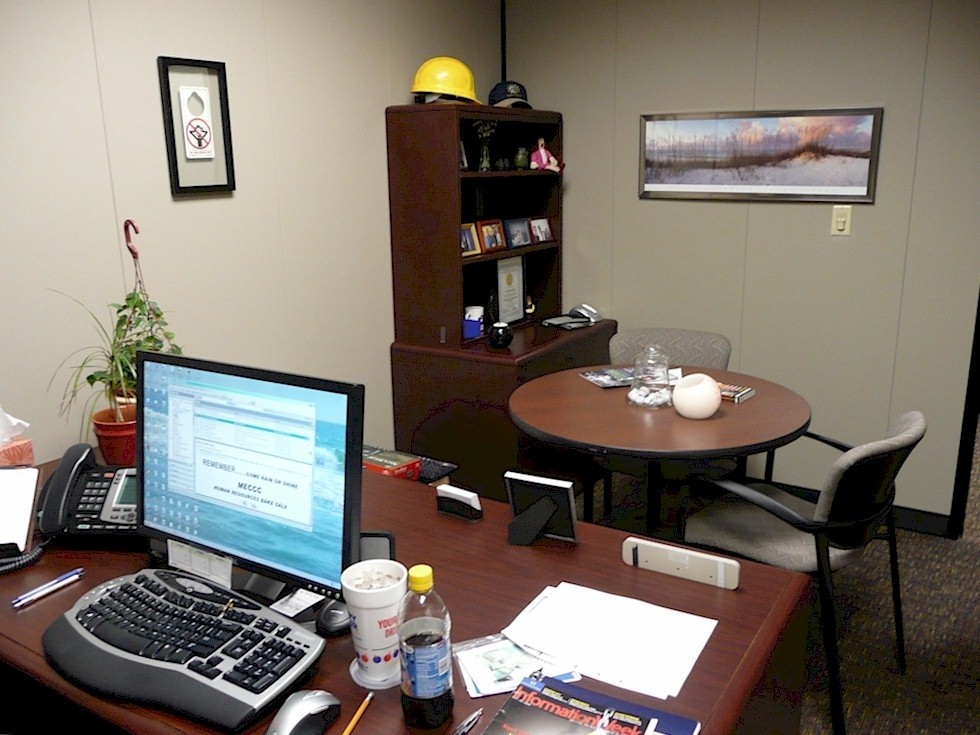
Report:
386,104,616,500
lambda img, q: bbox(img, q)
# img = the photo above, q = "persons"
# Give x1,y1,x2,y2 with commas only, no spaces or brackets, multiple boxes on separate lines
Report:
530,138,565,173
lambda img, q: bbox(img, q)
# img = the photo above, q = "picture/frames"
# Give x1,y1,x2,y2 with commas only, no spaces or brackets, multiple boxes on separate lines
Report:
157,55,236,194
638,107,884,205
475,219,506,254
461,223,481,257
503,218,532,250
528,216,554,244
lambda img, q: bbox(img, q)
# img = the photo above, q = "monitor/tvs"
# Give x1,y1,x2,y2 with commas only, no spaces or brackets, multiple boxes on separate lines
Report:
135,350,365,626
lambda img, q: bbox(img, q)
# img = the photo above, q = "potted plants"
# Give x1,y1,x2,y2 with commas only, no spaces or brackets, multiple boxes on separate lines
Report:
45,286,183,465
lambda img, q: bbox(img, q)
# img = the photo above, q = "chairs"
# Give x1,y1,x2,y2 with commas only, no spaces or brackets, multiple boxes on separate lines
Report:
608,328,732,370
676,409,927,735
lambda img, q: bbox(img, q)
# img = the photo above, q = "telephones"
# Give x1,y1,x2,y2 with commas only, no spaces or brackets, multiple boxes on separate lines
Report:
36,444,137,536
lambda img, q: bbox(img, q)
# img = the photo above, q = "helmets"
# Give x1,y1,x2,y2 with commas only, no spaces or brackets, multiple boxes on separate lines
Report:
413,56,484,106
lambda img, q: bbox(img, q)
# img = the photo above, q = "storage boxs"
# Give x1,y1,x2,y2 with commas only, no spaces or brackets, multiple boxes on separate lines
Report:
362,443,420,481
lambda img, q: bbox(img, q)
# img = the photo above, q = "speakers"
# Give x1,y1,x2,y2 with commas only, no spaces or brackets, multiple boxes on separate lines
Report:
359,531,397,562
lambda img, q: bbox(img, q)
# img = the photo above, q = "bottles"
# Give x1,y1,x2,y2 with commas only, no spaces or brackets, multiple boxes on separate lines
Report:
625,344,674,410
397,563,455,730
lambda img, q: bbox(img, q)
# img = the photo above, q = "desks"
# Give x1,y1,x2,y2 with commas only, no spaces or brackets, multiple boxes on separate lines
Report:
507,365,813,537
0,471,812,735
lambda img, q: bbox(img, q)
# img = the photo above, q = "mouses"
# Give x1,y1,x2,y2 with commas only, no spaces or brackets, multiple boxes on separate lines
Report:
263,689,342,735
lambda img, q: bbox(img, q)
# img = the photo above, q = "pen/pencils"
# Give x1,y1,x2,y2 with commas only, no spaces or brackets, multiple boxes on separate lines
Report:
342,692,375,735
14,571,86,607
11,568,83,604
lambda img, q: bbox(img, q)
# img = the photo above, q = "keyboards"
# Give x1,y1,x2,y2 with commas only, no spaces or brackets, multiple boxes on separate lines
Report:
40,565,328,732
362,444,458,483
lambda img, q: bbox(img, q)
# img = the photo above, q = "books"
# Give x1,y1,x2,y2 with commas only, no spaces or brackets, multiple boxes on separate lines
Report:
578,367,636,388
718,382,755,403
481,674,702,735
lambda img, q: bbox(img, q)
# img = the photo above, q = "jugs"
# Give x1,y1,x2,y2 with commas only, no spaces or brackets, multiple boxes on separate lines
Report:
514,147,528,171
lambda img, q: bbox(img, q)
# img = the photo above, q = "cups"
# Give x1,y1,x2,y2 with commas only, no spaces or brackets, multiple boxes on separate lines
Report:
340,559,408,681
465,306,484,332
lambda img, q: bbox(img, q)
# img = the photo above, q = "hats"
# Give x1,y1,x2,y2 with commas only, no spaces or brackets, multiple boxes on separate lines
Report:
489,81,533,109
